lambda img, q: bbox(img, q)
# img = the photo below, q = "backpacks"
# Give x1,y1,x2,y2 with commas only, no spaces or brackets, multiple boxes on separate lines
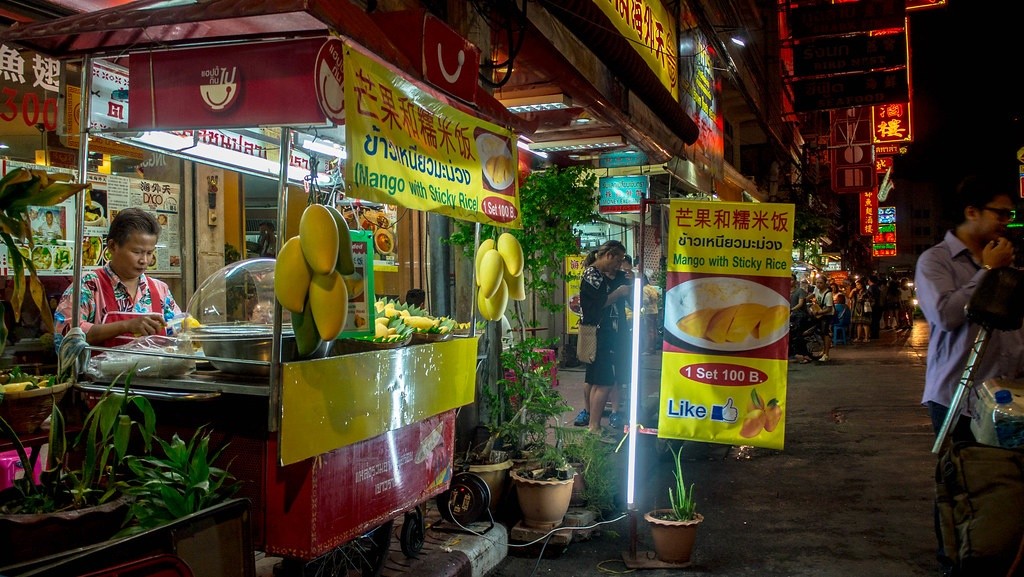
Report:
935,440,1024,577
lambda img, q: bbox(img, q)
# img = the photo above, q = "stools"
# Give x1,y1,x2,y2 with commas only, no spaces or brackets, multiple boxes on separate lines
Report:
833,326,847,346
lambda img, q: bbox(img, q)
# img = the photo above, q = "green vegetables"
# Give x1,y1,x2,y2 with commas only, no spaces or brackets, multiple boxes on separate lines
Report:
56,251,69,269
89,237,99,251
106,250,112,260
43,239,59,254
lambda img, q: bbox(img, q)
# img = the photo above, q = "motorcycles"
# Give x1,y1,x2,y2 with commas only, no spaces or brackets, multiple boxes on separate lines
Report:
790,307,827,360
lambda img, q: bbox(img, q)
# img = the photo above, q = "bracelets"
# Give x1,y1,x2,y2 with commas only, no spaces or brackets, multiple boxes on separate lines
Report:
984,264,993,271
816,311,819,315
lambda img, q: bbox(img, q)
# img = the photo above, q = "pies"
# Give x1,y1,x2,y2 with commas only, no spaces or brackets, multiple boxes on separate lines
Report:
85,212,98,221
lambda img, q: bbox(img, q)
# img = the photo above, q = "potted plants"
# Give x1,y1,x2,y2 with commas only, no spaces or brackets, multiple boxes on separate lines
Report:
646,439,709,563
0,368,76,435
0,356,148,564
454,153,612,524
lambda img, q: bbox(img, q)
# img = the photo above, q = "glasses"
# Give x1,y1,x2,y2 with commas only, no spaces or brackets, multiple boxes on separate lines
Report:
974,204,1015,223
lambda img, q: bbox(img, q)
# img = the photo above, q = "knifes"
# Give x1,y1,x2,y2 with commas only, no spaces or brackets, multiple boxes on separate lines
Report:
134,317,185,337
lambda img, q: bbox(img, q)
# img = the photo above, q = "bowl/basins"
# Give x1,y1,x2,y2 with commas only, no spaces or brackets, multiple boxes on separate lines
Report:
7,231,110,270
84,201,104,226
375,230,394,255
192,326,336,378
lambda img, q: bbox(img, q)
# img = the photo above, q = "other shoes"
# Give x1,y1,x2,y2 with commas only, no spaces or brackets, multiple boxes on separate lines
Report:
641,351,656,355
603,402,625,412
586,425,618,444
863,338,871,342
853,338,862,342
883,324,913,330
816,351,824,356
819,354,832,361
799,356,813,364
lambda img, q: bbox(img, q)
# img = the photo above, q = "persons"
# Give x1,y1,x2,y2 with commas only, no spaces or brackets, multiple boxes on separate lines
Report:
54,208,186,467
788,272,914,364
247,223,291,325
501,315,517,372
915,180,1024,577
574,240,660,445
28,206,63,240
405,289,426,311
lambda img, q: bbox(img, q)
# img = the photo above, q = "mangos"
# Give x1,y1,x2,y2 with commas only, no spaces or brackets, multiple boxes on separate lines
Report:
486,156,512,184
476,233,525,321
274,204,354,356
375,301,471,342
181,318,202,349
0,380,50,392
677,304,789,344
739,393,782,438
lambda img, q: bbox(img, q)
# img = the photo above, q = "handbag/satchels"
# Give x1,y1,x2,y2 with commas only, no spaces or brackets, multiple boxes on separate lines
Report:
819,291,840,324
864,301,873,313
576,317,601,364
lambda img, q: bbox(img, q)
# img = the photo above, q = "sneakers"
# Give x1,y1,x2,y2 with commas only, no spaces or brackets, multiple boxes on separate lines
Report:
574,408,590,426
609,412,623,428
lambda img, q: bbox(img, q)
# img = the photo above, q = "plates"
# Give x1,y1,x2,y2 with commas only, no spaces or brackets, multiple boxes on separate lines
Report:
665,277,791,351
569,295,581,315
477,133,514,190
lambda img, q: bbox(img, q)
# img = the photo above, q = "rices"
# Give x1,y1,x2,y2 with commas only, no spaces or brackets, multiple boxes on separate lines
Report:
682,279,748,309
482,136,505,155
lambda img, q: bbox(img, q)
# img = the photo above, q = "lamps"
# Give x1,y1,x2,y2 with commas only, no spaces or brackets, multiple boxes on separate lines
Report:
716,23,749,49
294,134,349,160
528,136,628,154
517,140,548,158
500,94,573,114
626,272,645,509
113,122,333,187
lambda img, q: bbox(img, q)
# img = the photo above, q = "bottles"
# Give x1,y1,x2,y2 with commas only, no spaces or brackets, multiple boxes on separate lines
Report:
992,389,1024,449
